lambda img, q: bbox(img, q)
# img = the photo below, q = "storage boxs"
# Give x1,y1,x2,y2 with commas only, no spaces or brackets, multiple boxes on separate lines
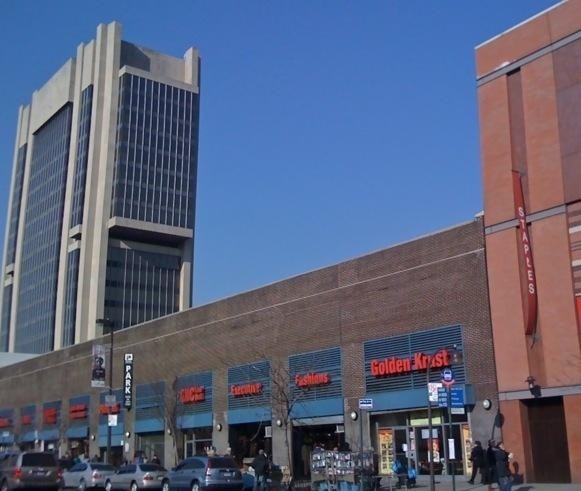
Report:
319,480,361,491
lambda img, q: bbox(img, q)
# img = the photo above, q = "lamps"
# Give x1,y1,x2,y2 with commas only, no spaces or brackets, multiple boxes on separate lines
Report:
525,376,540,398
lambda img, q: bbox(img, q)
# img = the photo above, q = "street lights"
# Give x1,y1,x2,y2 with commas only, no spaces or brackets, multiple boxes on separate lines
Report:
95,317,114,465
425,344,462,491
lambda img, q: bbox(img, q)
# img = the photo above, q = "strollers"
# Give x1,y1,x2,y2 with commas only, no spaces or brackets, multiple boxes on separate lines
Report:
392,459,416,488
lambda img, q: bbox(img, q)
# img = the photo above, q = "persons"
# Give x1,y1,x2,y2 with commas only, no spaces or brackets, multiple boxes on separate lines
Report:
92,357,106,381
467,441,486,485
300,439,352,476
91,454,101,463
487,440,499,491
151,456,161,465
495,440,515,491
252,450,270,491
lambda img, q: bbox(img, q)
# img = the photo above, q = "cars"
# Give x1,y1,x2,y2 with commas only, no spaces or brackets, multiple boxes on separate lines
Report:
62,462,116,490
0,451,64,491
102,463,166,490
160,455,243,491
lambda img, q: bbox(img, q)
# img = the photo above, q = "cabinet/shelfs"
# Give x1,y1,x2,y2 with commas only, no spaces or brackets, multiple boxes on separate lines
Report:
310,448,375,491
